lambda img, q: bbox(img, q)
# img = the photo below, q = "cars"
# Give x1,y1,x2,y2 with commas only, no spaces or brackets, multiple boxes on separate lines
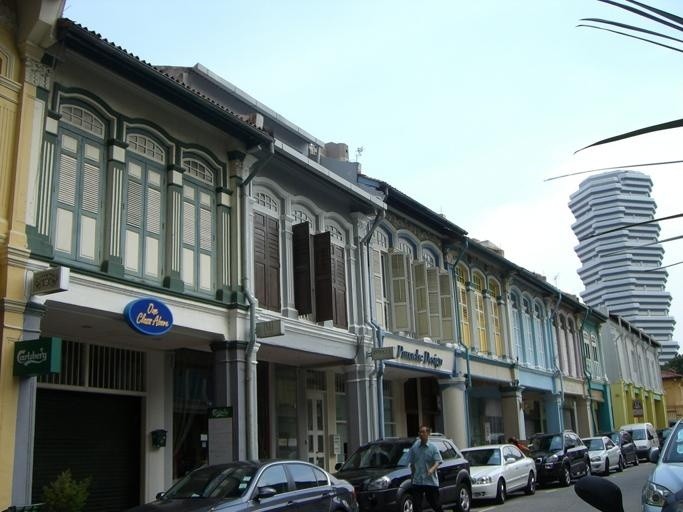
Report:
581,422,673,475
462,444,538,503
641,417,683,511
126,460,359,512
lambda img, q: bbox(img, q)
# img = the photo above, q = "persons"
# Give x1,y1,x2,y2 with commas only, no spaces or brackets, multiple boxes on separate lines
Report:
406,424,446,512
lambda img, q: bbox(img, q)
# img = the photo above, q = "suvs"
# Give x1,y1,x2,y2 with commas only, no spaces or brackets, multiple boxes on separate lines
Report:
334,433,473,511
527,430,592,487
